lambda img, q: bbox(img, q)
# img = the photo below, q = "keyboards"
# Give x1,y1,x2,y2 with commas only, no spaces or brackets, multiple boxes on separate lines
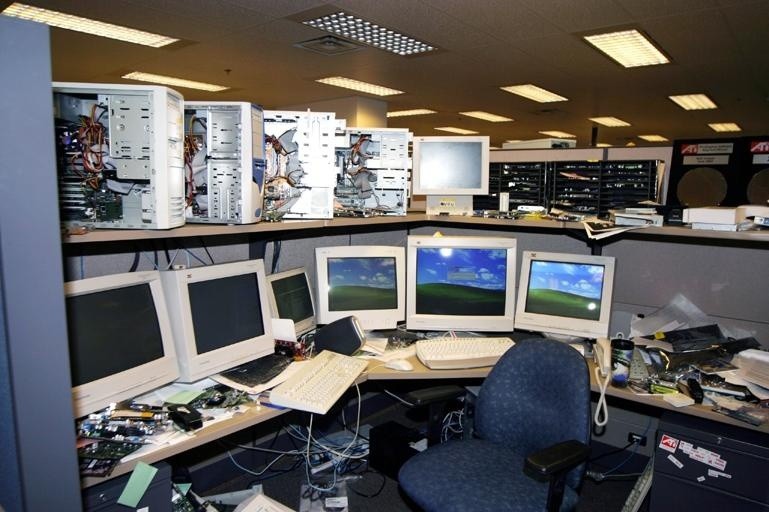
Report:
415,337,516,370
623,449,654,512
269,349,370,415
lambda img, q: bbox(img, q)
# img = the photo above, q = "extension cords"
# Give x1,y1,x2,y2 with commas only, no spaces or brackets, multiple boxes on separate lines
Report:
311,456,355,475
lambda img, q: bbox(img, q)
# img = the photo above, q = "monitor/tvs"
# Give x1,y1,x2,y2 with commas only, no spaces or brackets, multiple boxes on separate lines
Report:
315,245,405,329
266,266,316,341
160,258,275,383
406,235,517,339
412,136,490,216
64,269,181,420
513,250,617,343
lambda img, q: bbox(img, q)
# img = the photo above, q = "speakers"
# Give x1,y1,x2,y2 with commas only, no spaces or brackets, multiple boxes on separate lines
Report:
314,315,366,356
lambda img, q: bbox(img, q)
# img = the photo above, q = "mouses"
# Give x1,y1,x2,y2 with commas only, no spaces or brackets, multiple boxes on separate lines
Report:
384,358,414,371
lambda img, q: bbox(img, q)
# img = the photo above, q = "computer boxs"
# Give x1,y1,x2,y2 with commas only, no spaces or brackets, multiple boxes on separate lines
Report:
184,101,265,226
262,109,336,221
52,81,185,230
333,127,409,218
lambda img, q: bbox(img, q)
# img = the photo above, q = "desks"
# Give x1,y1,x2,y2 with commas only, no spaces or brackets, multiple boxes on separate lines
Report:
368,328,769,434
66,335,368,490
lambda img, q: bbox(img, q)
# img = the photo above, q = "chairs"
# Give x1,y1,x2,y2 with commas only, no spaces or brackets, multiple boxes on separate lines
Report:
397,335,592,512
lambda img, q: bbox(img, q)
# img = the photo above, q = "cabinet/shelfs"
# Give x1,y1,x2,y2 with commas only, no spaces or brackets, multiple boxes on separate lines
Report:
474,160,658,220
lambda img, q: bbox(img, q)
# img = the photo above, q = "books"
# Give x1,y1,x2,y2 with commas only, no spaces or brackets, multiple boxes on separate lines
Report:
682,206,746,224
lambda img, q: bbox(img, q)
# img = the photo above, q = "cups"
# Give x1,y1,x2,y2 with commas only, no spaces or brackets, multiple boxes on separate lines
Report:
609,339,635,388
